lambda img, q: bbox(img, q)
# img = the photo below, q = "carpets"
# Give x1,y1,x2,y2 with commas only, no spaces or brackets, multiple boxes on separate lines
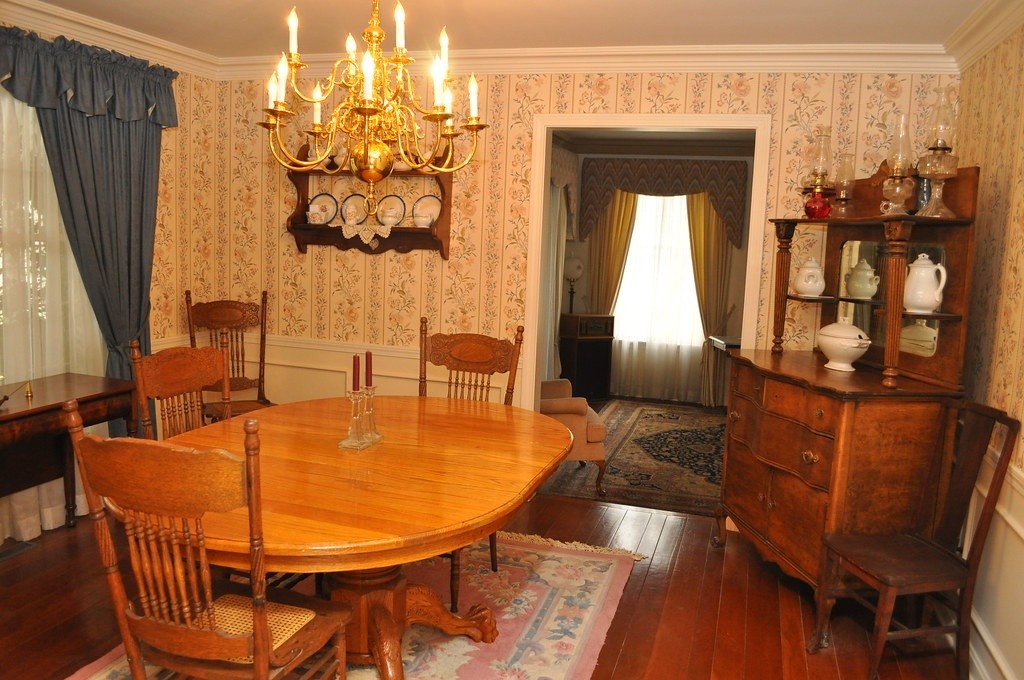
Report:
61,528,635,680
538,398,727,517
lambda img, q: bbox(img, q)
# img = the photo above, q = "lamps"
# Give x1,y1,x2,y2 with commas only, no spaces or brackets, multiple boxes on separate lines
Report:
913,138,961,218
803,135,833,218
256,0,491,218
566,250,585,312
879,114,917,217
832,152,857,217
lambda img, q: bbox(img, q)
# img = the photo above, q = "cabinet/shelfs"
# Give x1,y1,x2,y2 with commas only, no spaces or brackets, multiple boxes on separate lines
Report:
711,343,969,646
284,142,453,262
768,159,984,389
562,312,613,399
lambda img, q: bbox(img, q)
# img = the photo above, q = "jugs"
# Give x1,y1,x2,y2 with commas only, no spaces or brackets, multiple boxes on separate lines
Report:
903,253,946,312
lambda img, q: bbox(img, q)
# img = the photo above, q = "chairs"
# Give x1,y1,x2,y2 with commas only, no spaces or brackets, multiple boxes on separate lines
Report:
63,397,354,680
126,327,323,596
184,287,279,423
802,397,1021,680
540,378,611,499
418,314,529,609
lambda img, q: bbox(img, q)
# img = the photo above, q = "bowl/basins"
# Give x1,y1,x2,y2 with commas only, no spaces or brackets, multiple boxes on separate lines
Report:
816,317,871,371
901,318,939,356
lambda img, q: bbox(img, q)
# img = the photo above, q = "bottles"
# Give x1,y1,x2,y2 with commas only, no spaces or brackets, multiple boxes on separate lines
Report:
845,259,879,299
794,256,826,297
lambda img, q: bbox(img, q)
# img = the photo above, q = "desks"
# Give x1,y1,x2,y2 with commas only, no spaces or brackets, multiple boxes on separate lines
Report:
103,395,576,680
706,333,741,353
0,372,141,529
